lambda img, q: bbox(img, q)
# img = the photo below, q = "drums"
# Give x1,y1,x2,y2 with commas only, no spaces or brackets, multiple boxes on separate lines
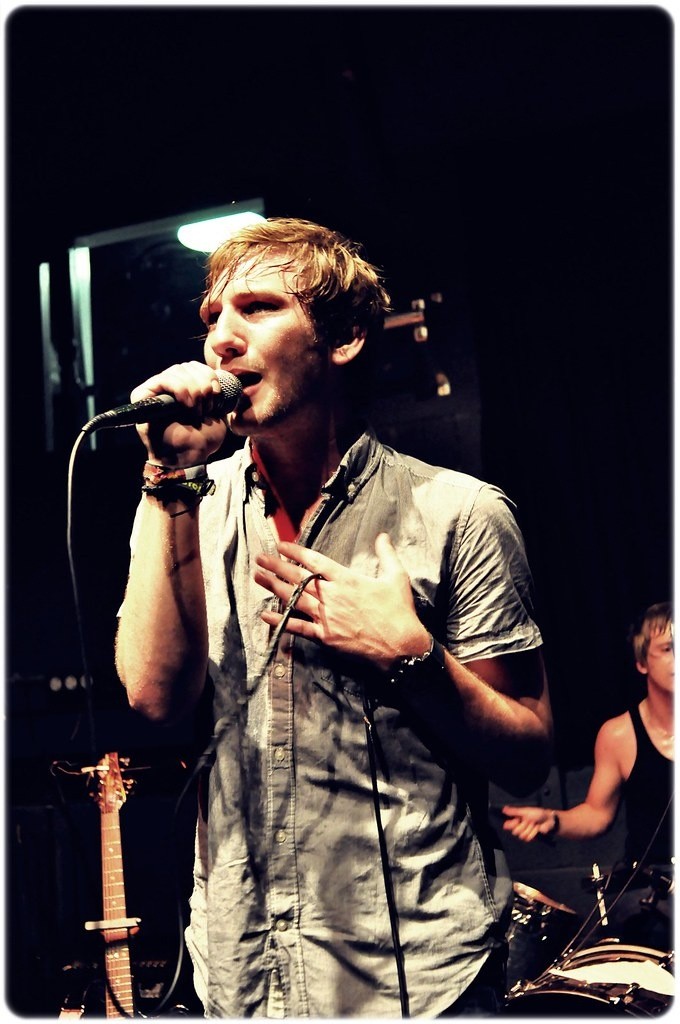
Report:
499,930,672,1017
482,870,579,1008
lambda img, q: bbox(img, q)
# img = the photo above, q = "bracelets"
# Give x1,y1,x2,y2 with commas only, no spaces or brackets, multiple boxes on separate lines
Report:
552,811,560,835
138,455,218,520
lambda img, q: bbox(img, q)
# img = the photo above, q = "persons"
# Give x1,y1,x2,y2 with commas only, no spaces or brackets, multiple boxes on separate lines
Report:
498,598,673,968
109,215,556,1019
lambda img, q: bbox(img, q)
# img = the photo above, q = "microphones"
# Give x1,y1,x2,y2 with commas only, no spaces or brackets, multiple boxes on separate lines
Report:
591,864,609,926
80,370,244,436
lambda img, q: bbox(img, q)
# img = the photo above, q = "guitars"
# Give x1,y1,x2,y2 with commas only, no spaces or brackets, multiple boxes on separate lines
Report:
41,745,195,1024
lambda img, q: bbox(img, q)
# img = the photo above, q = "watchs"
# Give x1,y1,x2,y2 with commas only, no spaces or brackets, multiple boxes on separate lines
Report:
383,630,447,703
